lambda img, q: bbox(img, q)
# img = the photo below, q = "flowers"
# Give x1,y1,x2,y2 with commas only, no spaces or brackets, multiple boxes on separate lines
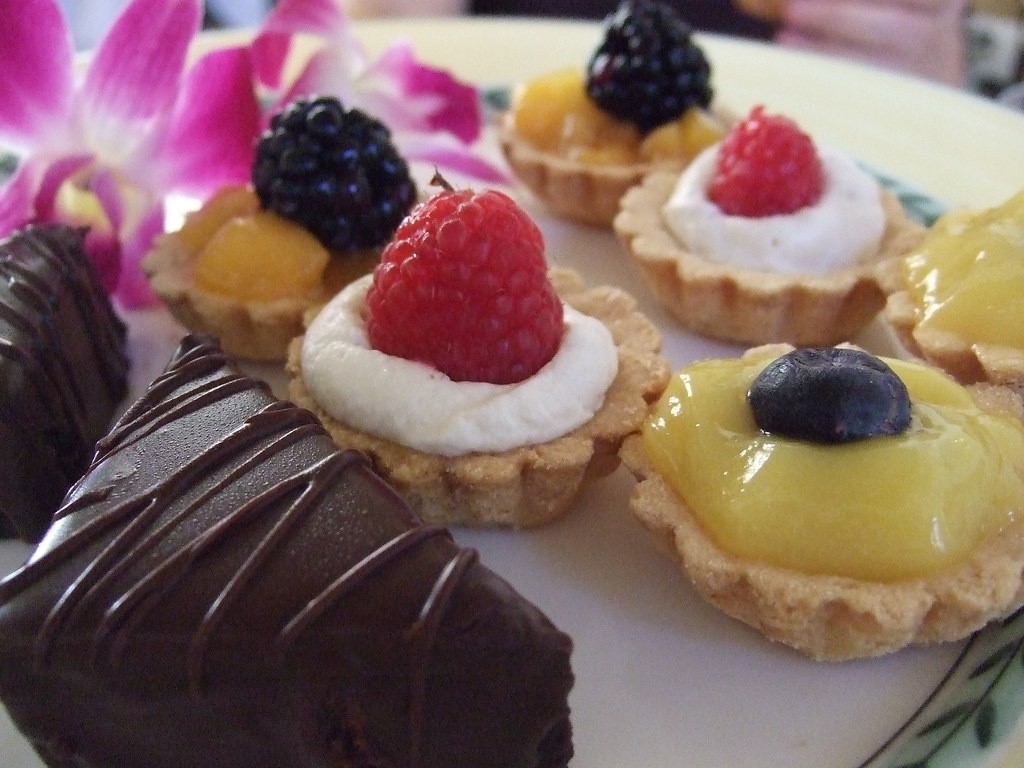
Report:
0,0,518,337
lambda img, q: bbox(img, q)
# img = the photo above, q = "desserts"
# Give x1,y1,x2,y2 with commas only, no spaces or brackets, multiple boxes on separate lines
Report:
0,0,1024,768
